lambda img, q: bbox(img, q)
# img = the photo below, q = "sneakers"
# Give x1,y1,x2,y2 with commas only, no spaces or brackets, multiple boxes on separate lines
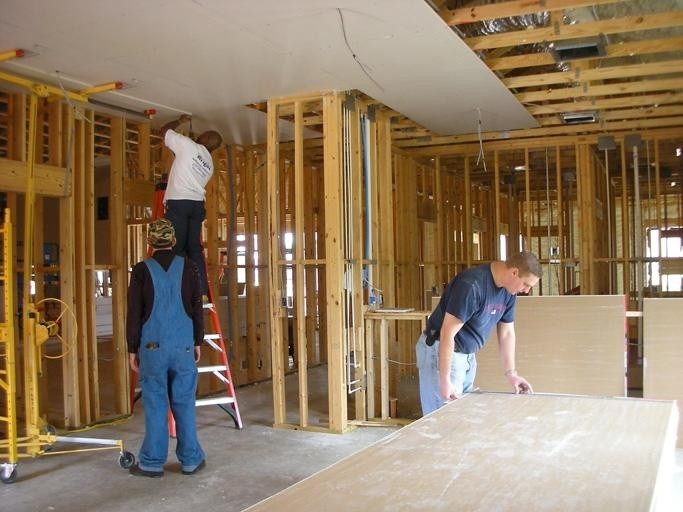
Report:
182,459,205,475
130,462,164,478
201,294,209,304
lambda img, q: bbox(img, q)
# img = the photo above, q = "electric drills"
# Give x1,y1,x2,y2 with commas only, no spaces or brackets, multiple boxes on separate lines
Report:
190,118,195,141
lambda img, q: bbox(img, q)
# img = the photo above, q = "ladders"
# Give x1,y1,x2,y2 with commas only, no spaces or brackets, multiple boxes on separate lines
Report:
128,182,243,438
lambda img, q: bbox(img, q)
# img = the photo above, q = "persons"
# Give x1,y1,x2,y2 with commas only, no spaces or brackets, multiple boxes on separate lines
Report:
123,217,207,479
412,251,545,420
157,113,222,296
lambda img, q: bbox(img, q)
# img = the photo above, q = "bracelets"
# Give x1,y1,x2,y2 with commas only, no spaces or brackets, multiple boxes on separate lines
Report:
505,368,517,377
175,119,183,126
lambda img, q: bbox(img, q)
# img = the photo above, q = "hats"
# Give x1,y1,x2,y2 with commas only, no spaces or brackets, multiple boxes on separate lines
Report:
148,219,175,249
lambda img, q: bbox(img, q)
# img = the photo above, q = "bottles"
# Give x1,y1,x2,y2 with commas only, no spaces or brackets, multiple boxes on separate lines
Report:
379,288,384,308
368,289,376,313
